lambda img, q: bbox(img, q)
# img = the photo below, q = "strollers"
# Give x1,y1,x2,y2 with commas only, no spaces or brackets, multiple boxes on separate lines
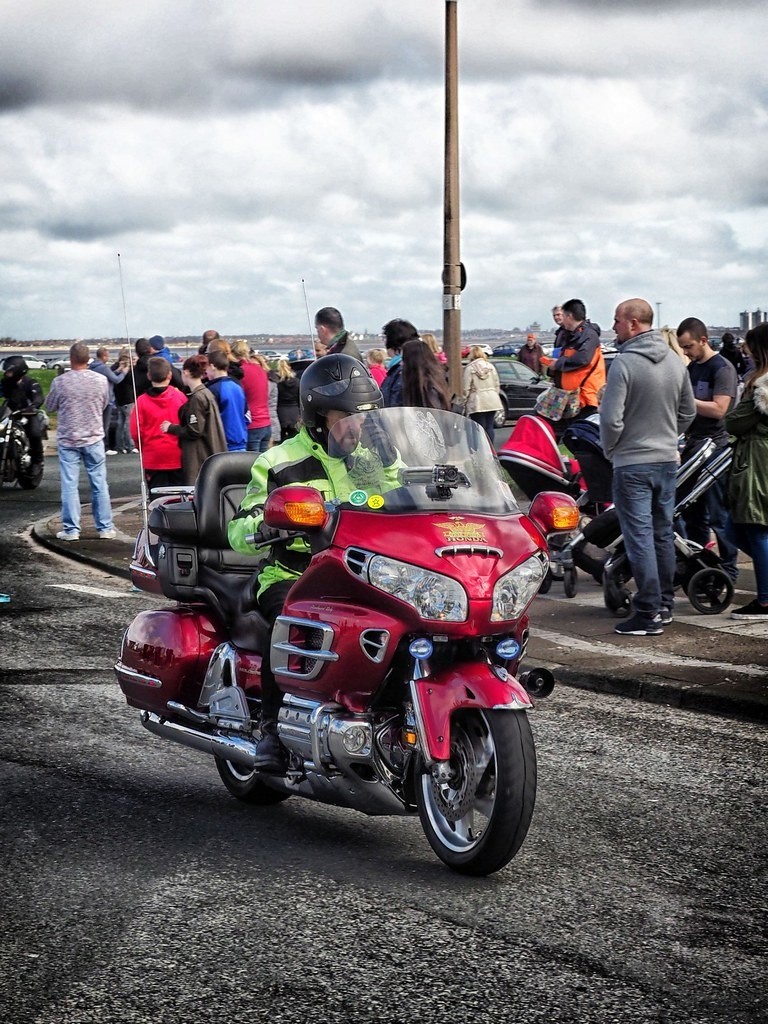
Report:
497,414,598,598
562,413,738,618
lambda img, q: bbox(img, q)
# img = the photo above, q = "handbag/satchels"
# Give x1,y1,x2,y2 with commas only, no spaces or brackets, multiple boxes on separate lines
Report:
534,384,582,421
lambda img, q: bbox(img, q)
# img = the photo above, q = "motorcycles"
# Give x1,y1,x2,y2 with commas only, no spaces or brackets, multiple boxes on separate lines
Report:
113,407,580,876
0,397,44,489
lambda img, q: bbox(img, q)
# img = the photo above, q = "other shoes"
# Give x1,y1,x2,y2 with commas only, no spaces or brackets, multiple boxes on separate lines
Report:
106,448,139,456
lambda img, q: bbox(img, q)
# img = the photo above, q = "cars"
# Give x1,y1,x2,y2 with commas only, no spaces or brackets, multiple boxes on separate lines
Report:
169,352,182,362
47,356,72,369
461,358,551,428
255,349,289,360
541,344,554,355
22,355,47,369
468,344,493,357
495,343,520,357
288,350,316,360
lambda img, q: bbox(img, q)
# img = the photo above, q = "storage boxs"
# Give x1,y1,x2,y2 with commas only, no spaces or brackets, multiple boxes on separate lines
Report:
115,606,222,717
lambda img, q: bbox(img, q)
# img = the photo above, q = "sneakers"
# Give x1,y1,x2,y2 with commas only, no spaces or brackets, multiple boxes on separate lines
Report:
614,613,665,635
27,464,42,476
100,530,116,538
254,717,286,770
657,606,672,626
731,599,768,619
56,531,79,540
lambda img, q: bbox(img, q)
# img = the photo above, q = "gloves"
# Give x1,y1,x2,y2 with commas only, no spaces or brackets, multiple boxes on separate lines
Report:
257,521,296,546
359,409,397,467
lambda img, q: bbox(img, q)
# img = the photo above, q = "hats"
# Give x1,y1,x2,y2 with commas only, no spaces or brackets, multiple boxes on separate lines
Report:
150,335,164,350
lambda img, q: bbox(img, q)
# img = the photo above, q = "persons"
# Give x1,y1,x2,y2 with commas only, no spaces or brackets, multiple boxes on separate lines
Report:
88,307,503,506
1,355,45,477
675,317,740,588
224,352,413,774
725,321,768,619
599,299,697,635
518,334,545,377
46,343,119,540
549,298,607,424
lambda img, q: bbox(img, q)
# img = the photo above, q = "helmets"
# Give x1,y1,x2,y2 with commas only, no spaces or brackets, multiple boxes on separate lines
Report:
300,353,384,427
3,356,28,382
